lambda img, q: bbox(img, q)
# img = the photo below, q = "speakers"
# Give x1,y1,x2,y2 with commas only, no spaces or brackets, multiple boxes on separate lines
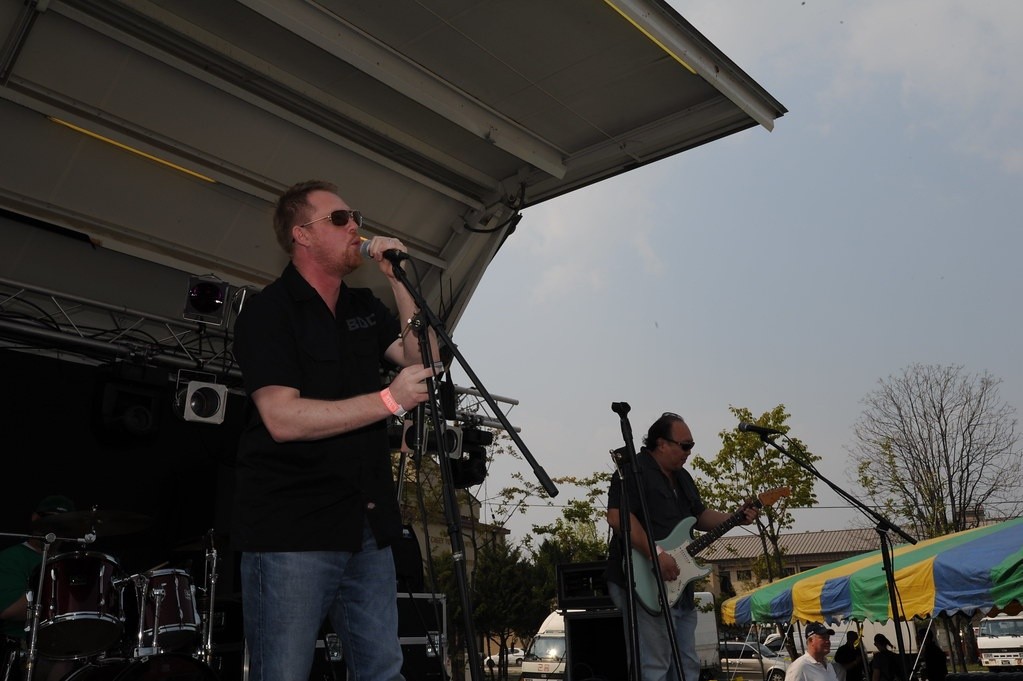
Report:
564,610,640,681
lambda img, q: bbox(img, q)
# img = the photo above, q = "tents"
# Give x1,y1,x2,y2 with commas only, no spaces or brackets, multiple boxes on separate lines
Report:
721,516,1023,681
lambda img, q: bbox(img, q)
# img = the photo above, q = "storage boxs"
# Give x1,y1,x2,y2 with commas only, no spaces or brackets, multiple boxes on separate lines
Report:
242,594,446,681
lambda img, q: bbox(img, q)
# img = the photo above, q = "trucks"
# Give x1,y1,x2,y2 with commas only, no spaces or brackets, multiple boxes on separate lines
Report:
762,618,917,657
522,591,721,681
977,613,1023,672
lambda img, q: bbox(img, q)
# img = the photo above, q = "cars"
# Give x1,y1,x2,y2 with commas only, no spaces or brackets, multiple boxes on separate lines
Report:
484,648,527,668
719,642,792,681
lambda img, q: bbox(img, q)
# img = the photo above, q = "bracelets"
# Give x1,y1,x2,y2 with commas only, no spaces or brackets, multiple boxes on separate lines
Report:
379,387,408,418
649,545,663,560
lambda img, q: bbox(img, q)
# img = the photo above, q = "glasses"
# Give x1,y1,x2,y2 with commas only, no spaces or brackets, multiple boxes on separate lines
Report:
663,437,695,451
291,210,362,242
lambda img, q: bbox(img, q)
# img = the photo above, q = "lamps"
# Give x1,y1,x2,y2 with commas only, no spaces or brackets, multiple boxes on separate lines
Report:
182,271,258,334
387,414,429,456
428,419,464,460
173,369,228,427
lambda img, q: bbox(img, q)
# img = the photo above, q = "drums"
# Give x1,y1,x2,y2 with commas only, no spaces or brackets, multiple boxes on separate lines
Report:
60,651,219,681
120,569,204,658
27,550,124,661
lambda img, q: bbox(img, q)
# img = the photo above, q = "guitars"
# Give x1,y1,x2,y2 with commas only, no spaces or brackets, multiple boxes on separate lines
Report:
623,483,790,616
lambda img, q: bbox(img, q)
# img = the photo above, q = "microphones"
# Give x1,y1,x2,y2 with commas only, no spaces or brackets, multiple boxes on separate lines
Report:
739,422,779,434
359,240,410,260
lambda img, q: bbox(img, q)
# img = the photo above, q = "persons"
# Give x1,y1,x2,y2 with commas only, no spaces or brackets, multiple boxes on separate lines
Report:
607,412,756,681
834,631,867,681
0,495,76,681
919,628,948,681
785,621,839,681
869,634,907,681
231,178,440,681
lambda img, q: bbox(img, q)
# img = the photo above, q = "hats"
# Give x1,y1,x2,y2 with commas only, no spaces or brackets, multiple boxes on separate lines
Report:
806,621,835,638
35,495,81,514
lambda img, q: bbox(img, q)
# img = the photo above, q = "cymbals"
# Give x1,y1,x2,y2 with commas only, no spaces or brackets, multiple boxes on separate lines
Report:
172,543,243,552
30,510,150,537
